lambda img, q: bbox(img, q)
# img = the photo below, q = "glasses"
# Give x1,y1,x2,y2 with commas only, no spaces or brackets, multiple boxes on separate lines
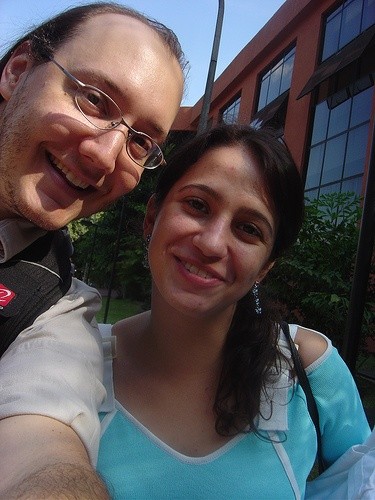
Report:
36,46,168,169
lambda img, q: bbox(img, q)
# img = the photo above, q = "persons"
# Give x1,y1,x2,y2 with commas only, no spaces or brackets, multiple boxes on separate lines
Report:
1,0,193,499
95,121,375,499
